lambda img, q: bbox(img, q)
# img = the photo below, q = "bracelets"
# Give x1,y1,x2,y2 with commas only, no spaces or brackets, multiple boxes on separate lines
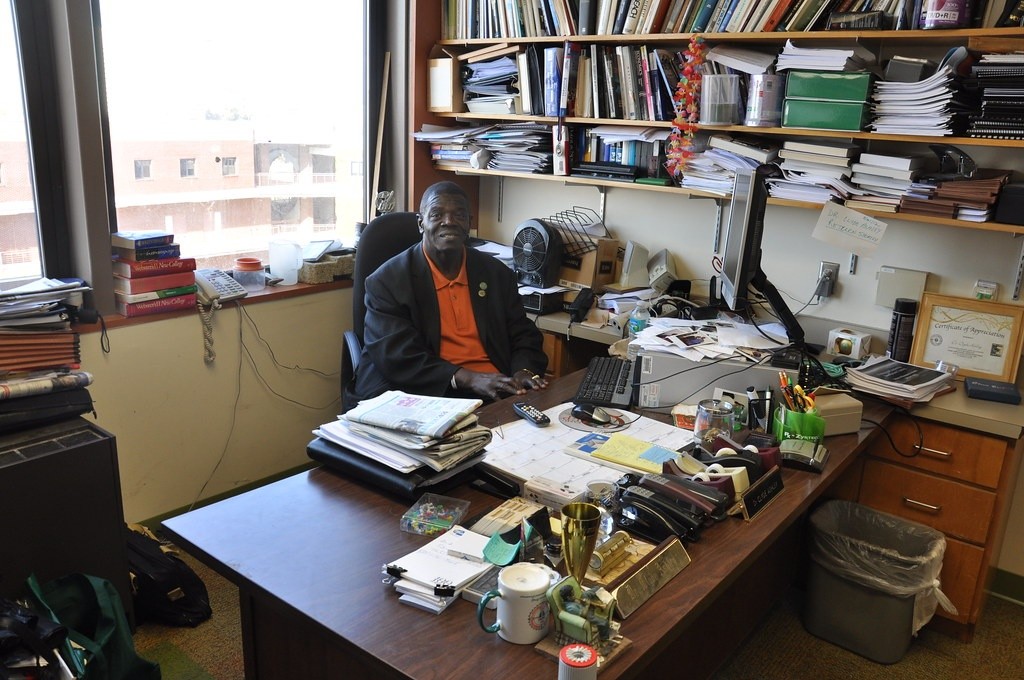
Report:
451,375,458,389
521,368,535,375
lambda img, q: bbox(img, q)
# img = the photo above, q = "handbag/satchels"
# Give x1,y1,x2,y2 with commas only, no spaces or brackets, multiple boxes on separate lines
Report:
15,564,162,680
123,526,212,628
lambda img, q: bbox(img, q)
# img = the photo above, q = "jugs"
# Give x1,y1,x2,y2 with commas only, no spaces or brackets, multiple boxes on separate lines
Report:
267,240,304,286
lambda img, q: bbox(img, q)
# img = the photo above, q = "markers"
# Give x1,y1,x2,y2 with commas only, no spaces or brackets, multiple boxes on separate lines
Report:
746,386,766,430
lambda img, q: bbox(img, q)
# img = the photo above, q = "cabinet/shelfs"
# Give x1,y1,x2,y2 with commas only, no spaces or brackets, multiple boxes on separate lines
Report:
831,413,1024,644
0,414,136,637
408,0,1024,392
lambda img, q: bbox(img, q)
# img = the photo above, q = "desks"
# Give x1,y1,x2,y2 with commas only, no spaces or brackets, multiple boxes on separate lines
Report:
161,363,894,680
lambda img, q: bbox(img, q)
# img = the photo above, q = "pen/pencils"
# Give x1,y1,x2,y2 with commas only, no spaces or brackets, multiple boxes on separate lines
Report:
765,385,770,433
779,369,795,411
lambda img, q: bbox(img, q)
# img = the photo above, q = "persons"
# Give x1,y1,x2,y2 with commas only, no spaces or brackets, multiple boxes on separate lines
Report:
363,179,550,405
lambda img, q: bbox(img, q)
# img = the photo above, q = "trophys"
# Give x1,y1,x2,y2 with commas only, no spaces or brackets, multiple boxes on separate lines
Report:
559,502,613,613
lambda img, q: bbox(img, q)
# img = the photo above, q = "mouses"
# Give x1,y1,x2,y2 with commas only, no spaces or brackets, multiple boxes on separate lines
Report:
571,403,611,425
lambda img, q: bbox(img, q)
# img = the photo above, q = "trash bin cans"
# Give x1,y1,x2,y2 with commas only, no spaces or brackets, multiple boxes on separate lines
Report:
803,499,943,664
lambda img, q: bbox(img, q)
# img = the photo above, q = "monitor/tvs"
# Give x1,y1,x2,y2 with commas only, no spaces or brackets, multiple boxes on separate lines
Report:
720,168,805,352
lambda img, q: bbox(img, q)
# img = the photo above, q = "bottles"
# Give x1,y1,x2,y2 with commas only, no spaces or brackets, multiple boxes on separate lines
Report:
886,297,920,363
232,258,265,291
626,301,650,362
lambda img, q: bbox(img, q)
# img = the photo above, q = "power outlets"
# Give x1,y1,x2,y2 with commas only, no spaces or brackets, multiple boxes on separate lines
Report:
818,261,840,295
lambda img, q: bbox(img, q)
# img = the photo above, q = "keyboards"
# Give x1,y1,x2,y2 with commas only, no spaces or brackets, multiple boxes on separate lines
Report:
573,355,636,410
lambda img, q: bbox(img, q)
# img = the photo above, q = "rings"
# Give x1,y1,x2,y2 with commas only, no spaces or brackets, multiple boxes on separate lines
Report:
532,374,540,379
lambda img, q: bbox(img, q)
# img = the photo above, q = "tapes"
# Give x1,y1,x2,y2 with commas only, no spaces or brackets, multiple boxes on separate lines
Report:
681,452,724,474
668,460,709,482
696,443,737,458
718,434,758,453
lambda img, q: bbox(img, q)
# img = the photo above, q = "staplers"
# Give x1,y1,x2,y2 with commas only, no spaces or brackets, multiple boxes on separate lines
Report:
609,473,729,547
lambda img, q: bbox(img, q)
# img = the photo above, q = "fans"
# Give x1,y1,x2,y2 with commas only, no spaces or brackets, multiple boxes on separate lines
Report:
512,219,563,289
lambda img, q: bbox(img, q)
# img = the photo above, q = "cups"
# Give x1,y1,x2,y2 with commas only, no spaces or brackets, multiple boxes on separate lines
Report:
745,75,784,127
583,479,620,548
559,503,602,585
693,399,735,453
700,74,740,125
477,563,550,645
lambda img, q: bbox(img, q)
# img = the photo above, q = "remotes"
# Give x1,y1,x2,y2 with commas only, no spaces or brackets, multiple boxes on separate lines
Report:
512,401,550,428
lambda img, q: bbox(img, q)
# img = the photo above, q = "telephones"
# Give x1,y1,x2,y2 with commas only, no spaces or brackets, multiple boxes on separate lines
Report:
193,268,248,306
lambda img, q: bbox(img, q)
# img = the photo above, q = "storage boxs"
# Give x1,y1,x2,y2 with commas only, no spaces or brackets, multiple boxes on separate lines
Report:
827,328,871,360
781,68,882,133
551,225,644,307
426,44,467,113
884,59,934,82
995,183,1024,227
523,476,584,512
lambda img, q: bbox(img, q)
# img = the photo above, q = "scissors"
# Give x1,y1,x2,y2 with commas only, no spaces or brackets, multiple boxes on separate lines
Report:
785,384,815,413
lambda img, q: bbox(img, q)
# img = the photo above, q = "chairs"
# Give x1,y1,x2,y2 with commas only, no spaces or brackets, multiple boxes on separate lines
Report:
342,211,423,415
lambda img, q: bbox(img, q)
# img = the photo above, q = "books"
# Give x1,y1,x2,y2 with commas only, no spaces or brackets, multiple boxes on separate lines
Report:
441,0,924,41
456,43,1024,138
678,133,1006,220
306,437,485,507
111,229,198,318
562,431,682,479
381,525,503,614
413,117,672,199
